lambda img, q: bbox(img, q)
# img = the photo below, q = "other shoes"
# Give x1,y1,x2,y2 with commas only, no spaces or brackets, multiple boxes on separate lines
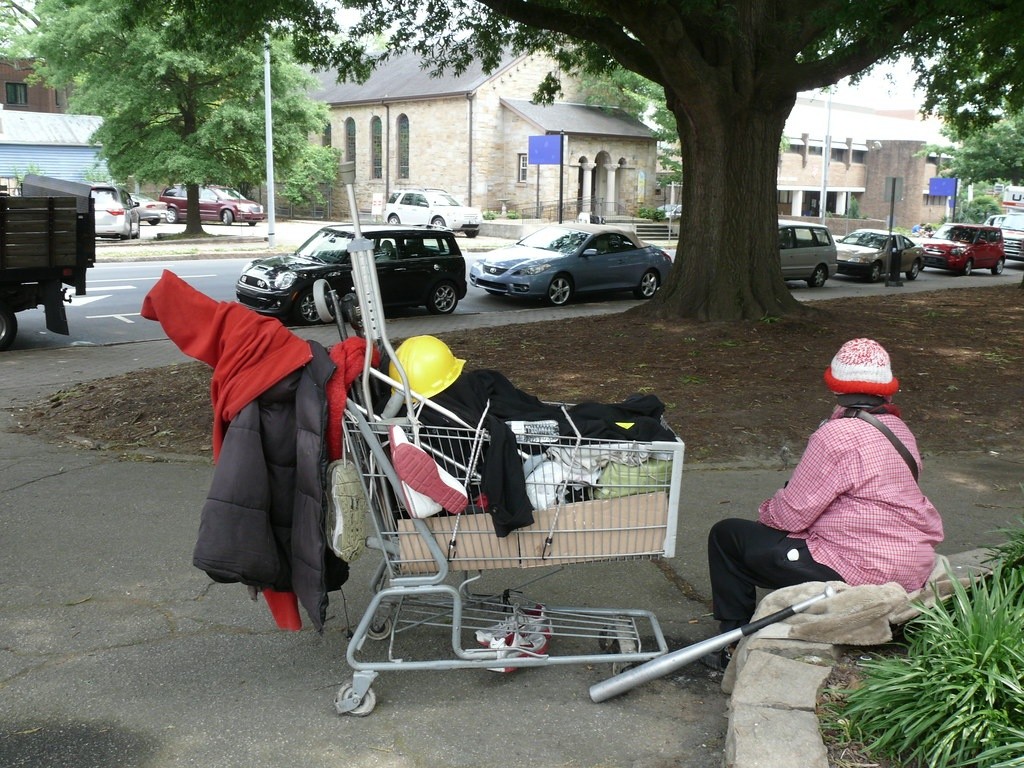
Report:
700,645,733,673
323,459,367,562
389,423,469,520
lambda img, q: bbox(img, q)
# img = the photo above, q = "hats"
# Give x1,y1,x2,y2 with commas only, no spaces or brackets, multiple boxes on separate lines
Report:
824,337,900,394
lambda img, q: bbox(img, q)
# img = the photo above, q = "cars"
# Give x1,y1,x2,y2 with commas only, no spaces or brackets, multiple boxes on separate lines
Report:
87,181,141,241
127,192,169,226
987,209,1024,264
834,228,927,283
159,181,266,227
468,220,675,307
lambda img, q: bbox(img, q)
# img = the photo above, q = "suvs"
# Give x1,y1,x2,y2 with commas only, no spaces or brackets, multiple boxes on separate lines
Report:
235,224,467,327
777,219,839,288
384,186,485,239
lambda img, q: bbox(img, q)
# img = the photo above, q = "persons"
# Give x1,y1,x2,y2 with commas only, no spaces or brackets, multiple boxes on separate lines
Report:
911,223,933,239
698,339,945,670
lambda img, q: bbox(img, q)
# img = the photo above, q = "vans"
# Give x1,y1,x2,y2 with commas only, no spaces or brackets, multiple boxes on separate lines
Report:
918,222,1006,277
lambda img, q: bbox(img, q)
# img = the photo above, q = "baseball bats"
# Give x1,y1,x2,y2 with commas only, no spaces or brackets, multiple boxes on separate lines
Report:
588,585,834,704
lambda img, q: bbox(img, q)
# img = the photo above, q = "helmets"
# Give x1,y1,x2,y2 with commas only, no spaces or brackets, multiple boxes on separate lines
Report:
389,334,466,404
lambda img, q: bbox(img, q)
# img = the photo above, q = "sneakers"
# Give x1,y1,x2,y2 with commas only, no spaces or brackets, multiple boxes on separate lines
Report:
473,603,553,673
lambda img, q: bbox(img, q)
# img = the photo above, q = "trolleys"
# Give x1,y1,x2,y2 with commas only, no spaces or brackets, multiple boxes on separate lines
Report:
333,367,686,719
